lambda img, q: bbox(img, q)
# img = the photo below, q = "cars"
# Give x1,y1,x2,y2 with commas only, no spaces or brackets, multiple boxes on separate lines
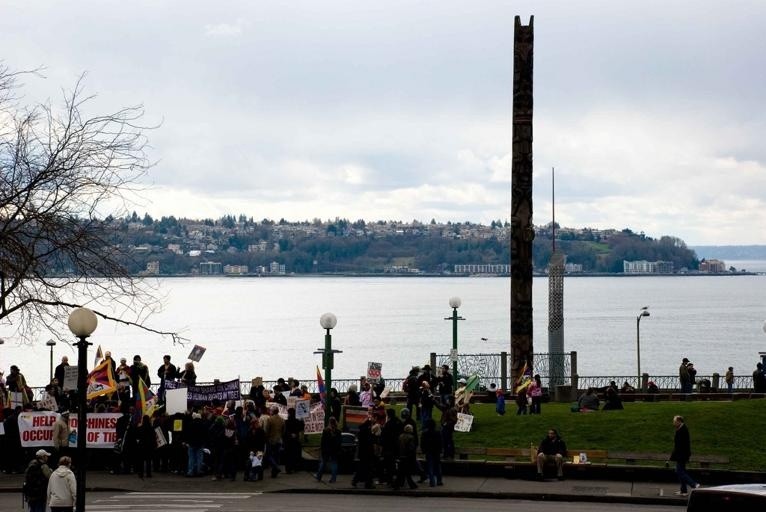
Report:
683,484,766,512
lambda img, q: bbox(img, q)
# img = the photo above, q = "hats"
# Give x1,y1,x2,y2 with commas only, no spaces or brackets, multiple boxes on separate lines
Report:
456,378,468,384
421,364,433,371
34,448,52,458
681,357,690,363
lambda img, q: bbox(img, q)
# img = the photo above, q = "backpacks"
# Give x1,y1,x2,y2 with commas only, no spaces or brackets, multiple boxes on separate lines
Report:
22,462,49,498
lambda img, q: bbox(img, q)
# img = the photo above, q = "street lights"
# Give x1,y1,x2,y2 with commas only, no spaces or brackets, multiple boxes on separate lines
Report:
313,312,343,401
46,335,58,397
632,303,651,390
63,303,101,511
442,292,467,395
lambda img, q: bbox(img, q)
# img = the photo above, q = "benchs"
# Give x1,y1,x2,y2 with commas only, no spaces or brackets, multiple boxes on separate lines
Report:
441,445,731,477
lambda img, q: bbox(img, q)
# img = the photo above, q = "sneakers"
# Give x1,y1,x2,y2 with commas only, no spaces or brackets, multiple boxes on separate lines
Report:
673,491,689,497
